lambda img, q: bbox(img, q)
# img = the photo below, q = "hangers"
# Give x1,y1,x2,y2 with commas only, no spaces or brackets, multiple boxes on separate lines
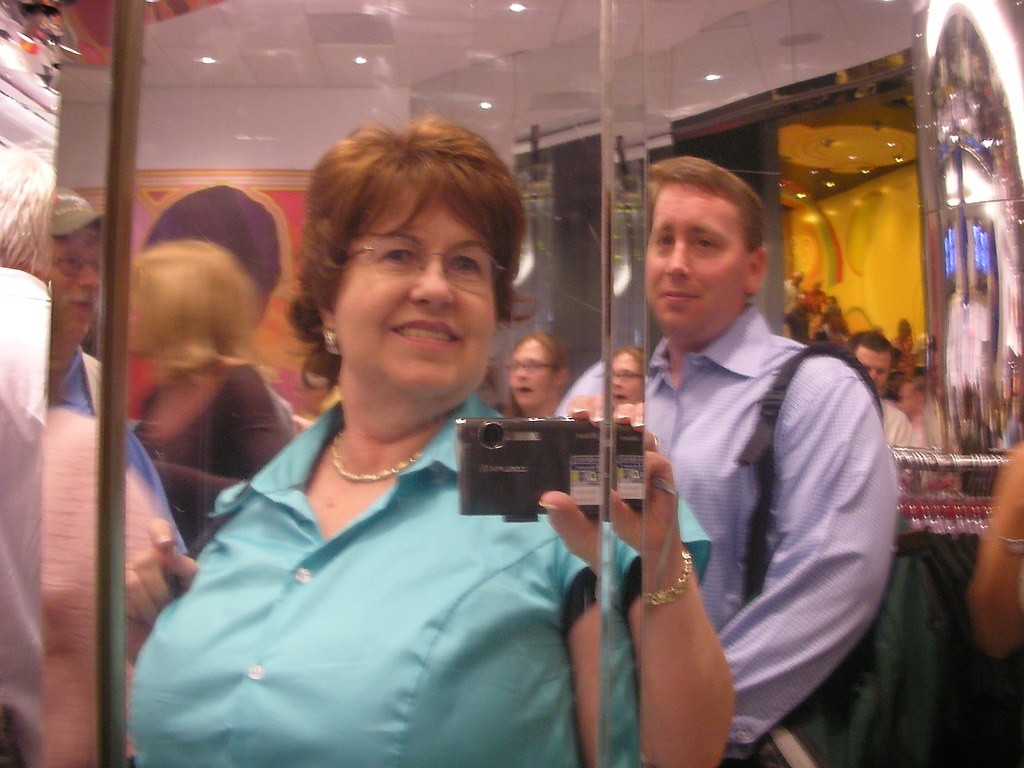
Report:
889,446,1011,539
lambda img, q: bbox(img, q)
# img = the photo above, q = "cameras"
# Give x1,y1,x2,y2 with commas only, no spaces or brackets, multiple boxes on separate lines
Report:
455,417,644,523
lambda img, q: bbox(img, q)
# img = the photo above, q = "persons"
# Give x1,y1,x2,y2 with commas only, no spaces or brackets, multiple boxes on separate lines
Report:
126,119,731,768
611,345,646,418
969,443,1024,669
643,157,897,764
848,332,917,451
0,143,290,768
507,332,570,423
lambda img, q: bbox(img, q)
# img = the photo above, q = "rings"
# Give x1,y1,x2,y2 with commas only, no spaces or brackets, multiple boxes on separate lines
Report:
652,435,660,446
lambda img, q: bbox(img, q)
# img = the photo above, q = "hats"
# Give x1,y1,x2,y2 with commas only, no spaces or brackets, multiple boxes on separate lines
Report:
52,189,105,234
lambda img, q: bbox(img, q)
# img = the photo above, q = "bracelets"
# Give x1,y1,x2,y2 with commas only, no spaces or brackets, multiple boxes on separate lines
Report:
634,550,693,609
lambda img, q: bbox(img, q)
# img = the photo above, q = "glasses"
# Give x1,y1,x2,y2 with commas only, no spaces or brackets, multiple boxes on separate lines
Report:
348,236,506,287
598,369,645,382
506,362,562,380
49,249,102,278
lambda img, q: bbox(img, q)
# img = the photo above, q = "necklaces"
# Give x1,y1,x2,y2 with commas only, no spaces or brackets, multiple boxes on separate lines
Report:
333,427,424,482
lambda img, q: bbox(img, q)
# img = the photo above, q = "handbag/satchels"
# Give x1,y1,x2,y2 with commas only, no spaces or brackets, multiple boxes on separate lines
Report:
723,344,940,768
